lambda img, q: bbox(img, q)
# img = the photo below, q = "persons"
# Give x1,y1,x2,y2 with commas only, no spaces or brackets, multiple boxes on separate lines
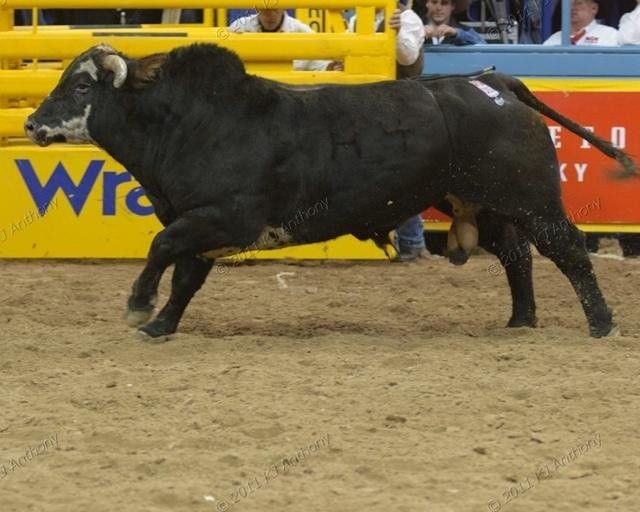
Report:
617,1,639,45
420,0,487,46
324,0,426,263
540,1,618,46
229,7,333,71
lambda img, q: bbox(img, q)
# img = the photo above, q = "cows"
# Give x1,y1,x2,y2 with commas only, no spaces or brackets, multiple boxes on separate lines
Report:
23,42,640,342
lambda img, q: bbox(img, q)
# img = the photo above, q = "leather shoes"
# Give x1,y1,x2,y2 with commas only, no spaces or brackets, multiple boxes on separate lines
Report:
392,246,422,263
